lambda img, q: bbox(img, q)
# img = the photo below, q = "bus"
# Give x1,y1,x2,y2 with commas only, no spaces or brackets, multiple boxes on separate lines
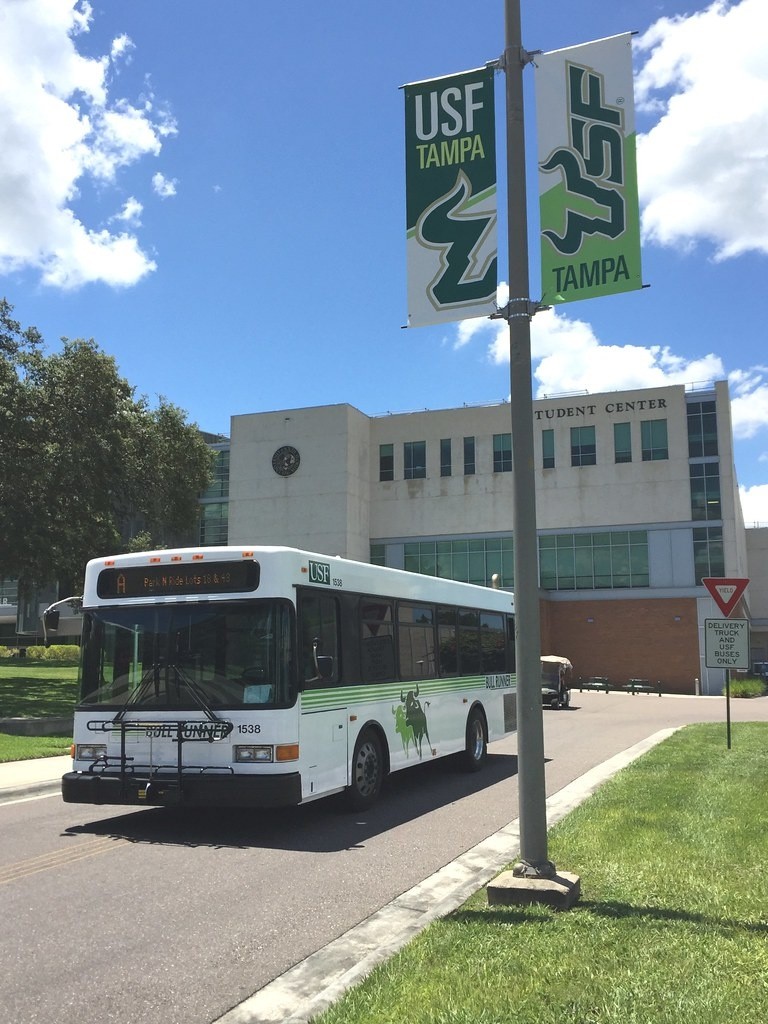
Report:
41,546,517,813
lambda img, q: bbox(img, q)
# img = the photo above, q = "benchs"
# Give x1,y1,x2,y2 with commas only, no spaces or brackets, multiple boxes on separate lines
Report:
582,683,614,688
623,684,655,690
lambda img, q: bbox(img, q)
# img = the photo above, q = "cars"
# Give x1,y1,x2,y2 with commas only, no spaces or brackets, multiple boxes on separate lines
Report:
582,676,615,691
622,678,654,693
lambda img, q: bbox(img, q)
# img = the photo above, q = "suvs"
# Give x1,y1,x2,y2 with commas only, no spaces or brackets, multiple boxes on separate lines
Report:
0,605,18,624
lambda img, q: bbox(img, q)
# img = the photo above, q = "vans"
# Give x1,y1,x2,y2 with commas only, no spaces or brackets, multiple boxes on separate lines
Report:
752,662,768,680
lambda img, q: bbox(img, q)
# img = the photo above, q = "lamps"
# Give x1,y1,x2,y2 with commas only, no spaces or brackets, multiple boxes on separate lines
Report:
675,616,681,620
587,618,594,622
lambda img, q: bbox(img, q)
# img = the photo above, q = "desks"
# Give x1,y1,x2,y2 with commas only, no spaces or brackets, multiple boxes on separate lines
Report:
586,676,609,692
629,679,649,696
